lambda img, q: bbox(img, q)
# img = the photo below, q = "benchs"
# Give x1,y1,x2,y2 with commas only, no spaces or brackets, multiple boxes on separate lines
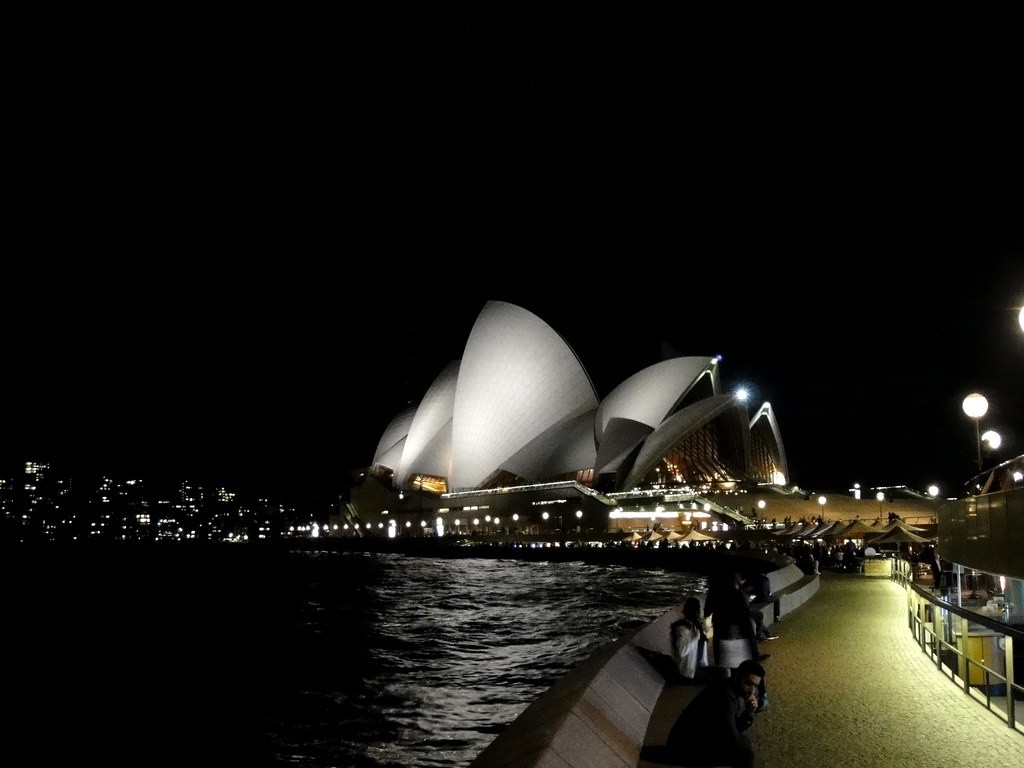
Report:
533,564,820,768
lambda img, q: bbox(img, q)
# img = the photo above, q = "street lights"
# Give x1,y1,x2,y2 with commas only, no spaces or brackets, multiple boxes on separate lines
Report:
963,393,1003,474
929,486,938,517
819,496,826,519
576,510,583,533
485,515,491,536
758,500,766,518
876,492,885,522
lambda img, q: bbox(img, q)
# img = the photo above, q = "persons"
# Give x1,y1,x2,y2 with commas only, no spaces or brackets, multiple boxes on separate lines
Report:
670,563,784,713
581,537,940,575
664,659,765,768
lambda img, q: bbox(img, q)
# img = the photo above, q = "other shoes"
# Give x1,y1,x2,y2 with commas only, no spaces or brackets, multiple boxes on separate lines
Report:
755,634,767,642
774,616,781,622
765,634,779,641
760,654,771,661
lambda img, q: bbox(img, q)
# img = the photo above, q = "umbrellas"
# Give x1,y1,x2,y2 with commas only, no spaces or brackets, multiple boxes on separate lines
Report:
772,515,930,549
623,529,716,542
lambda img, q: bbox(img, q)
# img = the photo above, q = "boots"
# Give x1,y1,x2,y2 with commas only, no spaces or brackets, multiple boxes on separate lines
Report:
754,692,769,713
